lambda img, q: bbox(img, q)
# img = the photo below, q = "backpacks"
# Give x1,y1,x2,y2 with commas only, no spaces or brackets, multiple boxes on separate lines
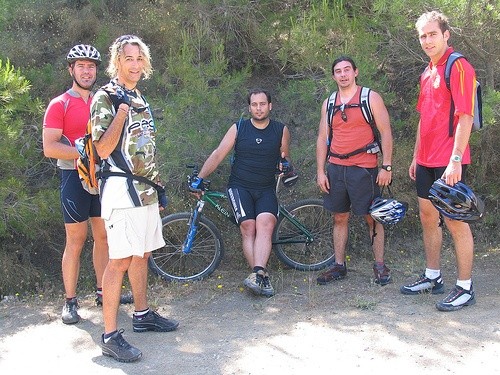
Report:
74,84,163,196
444,52,482,132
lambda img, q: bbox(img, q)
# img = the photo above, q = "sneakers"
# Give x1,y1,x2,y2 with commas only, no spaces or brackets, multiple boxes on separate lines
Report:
245,270,274,297
435,280,476,312
97,294,134,307
317,261,346,284
132,307,178,332
373,265,391,286
61,298,78,324
400,272,445,294
101,329,141,363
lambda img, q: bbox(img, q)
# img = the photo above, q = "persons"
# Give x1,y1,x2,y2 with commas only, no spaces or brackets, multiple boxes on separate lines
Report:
89,35,179,364
190,89,289,296
43,45,134,324
314,57,393,283
400,11,475,310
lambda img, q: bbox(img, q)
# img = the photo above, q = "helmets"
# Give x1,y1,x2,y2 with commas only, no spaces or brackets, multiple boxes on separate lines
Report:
67,43,102,64
426,178,479,220
369,196,408,226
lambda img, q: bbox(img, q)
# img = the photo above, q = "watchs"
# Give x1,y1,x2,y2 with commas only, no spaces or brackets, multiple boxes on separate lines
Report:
382,165,392,171
450,155,462,162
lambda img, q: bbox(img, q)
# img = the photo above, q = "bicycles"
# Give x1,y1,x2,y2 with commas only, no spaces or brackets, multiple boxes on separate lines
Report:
147,164,350,284
